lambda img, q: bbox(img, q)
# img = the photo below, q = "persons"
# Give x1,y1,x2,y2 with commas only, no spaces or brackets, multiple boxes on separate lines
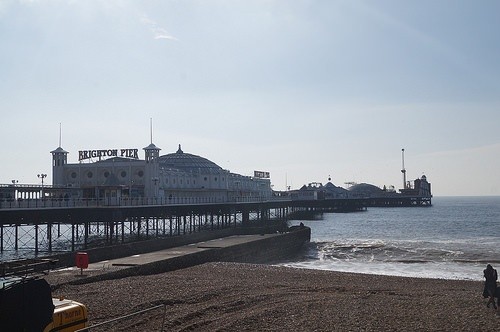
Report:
483,263,498,310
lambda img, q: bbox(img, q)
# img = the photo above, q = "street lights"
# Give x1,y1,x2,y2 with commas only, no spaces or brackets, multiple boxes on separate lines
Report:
37,173,48,208
